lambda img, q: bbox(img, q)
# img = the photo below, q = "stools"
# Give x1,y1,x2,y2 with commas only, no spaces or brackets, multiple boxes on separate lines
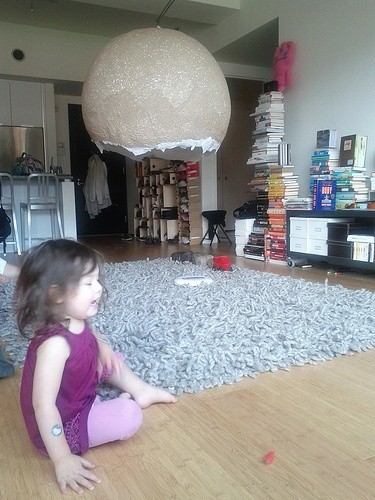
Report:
19,173,66,252
0,173,22,256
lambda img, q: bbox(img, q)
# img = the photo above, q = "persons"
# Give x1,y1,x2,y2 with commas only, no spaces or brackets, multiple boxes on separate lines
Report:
14,238,178,494
0,257,20,284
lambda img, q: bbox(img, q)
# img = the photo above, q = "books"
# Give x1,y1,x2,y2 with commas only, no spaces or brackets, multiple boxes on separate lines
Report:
308,129,375,211
244,91,300,261
285,198,312,210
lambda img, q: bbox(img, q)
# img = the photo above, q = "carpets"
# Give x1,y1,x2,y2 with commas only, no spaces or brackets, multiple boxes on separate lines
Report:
0,256,375,405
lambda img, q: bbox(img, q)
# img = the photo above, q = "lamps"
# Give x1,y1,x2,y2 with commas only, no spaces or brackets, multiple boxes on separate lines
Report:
81,1,231,162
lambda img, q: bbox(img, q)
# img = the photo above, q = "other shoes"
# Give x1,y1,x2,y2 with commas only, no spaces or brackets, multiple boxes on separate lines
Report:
138,235,147,241
121,232,133,241
182,222,189,227
180,197,188,203
182,236,190,245
179,204,188,213
144,238,160,244
178,172,186,179
178,180,187,187
177,164,186,171
167,236,179,243
180,215,189,221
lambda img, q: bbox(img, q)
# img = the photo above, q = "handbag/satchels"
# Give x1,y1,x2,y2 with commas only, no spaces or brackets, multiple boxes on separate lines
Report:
233,199,257,218
0,205,11,243
13,152,44,176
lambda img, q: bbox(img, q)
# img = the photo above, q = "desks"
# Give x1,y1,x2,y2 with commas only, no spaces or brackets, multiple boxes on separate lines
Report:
235,216,255,256
199,210,234,247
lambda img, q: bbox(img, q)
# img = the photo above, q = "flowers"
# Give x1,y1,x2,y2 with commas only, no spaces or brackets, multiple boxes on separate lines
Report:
9,151,44,177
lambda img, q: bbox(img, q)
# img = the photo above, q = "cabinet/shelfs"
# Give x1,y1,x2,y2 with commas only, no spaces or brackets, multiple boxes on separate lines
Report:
133,157,203,245
286,208,375,274
0,78,42,127
